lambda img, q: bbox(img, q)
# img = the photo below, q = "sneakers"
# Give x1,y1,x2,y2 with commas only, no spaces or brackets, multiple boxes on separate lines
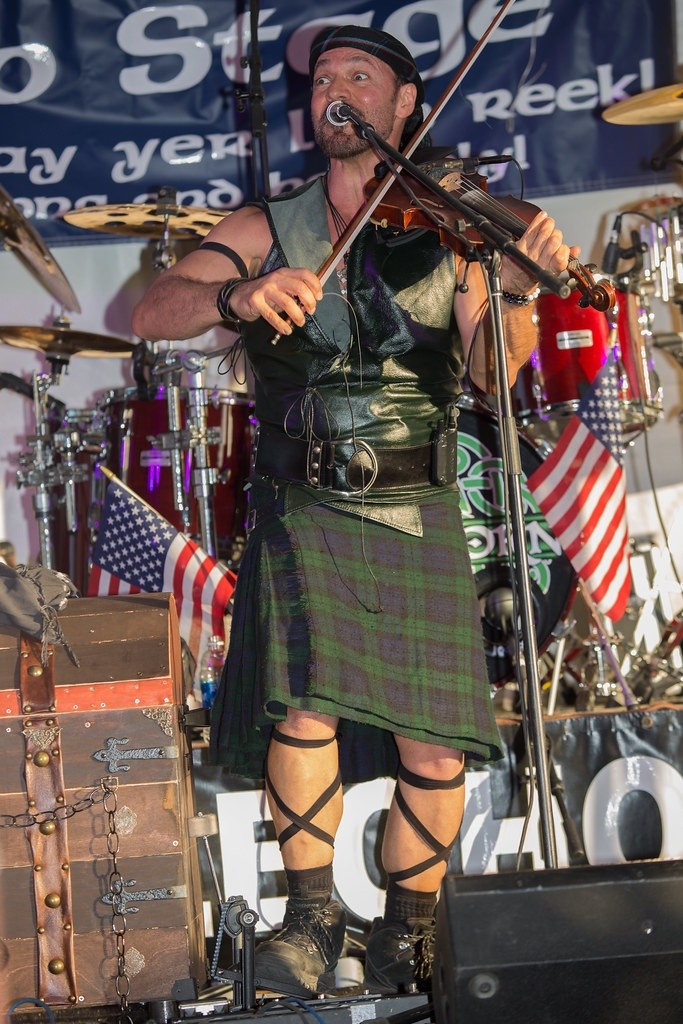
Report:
363,917,437,991
252,896,348,994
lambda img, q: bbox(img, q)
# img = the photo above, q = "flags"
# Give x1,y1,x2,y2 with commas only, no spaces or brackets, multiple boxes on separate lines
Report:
87,481,237,708
525,346,633,623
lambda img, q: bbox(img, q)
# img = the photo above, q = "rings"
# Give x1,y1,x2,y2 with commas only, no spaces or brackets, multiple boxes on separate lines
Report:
545,263,561,277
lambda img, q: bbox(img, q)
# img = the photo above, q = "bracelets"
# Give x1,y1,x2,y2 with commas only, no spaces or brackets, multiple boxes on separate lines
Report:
216,277,253,323
200,240,251,284
501,288,541,305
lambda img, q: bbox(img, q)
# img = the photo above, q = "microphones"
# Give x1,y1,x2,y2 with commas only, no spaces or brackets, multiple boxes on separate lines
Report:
326,100,355,127
602,215,622,275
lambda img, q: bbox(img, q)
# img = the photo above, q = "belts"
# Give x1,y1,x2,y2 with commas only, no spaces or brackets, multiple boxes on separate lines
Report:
251,418,459,493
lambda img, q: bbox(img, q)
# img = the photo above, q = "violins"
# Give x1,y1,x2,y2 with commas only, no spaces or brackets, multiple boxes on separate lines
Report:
364,147,617,309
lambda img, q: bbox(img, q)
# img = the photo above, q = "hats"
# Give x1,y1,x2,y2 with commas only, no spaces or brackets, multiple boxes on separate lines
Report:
309,24,426,105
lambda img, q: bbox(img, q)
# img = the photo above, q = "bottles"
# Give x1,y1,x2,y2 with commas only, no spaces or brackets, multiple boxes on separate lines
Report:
199,634,227,725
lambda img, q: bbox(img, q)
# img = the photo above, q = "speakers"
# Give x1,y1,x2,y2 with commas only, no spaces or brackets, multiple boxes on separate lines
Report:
433,857,683,1024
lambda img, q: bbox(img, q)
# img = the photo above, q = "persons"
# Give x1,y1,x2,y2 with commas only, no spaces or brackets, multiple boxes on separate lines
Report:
129,25,582,1000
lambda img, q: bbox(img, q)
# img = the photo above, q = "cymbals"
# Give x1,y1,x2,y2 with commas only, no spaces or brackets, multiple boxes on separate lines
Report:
1,325,137,360
66,204,233,238
602,82,683,126
620,197,683,214
0,183,81,313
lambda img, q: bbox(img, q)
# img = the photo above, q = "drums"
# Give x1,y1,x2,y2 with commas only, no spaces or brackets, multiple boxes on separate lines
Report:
506,275,664,443
43,434,100,597
90,384,259,567
455,391,579,691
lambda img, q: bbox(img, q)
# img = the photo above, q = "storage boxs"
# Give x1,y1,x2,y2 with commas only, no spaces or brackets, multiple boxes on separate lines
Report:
0,590,211,1024
